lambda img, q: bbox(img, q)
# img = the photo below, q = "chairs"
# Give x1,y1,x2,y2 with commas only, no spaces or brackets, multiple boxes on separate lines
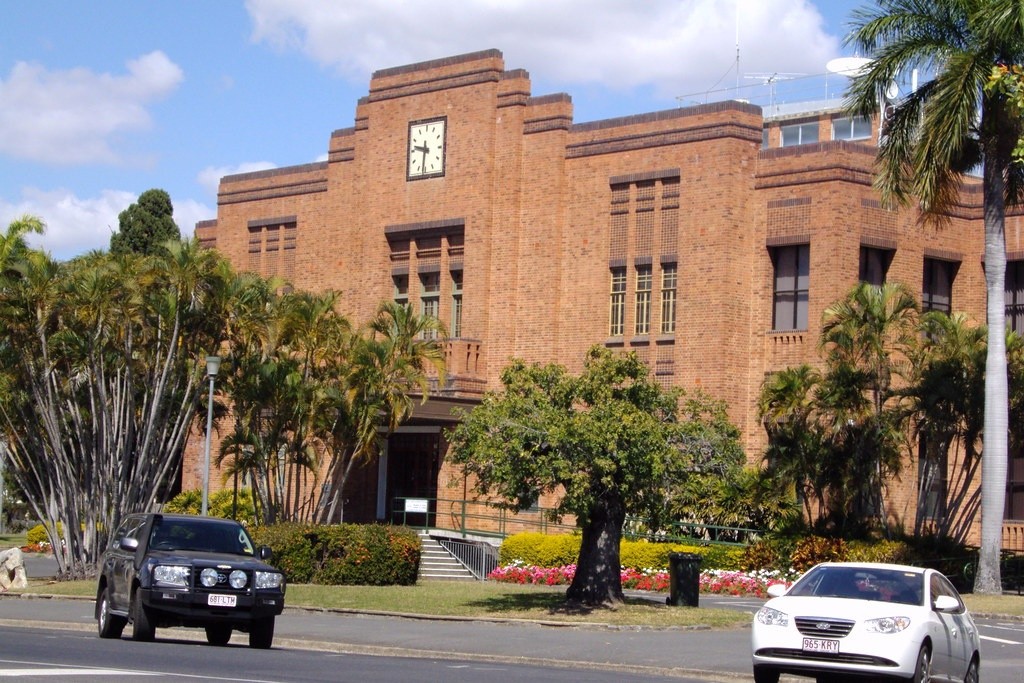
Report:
834,580,859,596
890,586,918,602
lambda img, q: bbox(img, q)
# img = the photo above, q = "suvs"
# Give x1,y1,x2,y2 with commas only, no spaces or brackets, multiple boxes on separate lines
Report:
94,513,286,649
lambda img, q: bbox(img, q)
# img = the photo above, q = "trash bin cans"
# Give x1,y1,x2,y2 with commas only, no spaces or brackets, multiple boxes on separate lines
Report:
665,550,702,608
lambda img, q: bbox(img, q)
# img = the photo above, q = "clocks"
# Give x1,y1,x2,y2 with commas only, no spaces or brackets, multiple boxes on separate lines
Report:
406,115,448,181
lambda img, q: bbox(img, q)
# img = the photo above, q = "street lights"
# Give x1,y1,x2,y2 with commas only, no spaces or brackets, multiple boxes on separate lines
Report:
202,356,222,516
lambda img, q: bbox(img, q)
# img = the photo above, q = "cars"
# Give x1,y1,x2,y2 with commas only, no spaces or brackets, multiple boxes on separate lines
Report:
750,562,981,683
